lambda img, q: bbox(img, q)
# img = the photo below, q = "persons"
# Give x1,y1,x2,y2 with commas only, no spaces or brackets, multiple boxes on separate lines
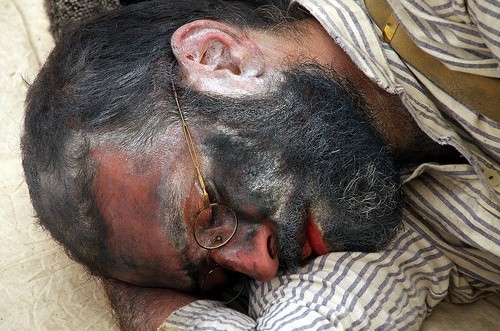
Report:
23,0,500,331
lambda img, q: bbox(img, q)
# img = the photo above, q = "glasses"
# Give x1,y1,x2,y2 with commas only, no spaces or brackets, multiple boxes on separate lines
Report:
169,60,247,305
